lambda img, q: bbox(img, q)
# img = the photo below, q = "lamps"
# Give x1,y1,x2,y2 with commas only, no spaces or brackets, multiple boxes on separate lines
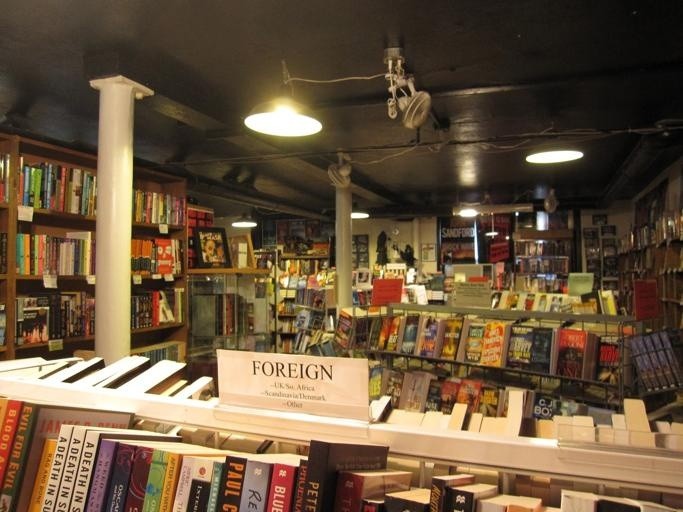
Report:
243,84,322,137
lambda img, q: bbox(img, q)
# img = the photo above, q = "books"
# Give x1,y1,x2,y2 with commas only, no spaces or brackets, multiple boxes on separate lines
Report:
190,293,255,335
131,287,187,328
617,211,679,331
131,239,184,275
0,291,94,346
514,241,572,256
0,394,680,511
259,254,632,440
2,355,220,406
3,159,96,215
132,189,186,226
631,331,680,392
583,225,617,256
130,340,185,361
2,231,95,276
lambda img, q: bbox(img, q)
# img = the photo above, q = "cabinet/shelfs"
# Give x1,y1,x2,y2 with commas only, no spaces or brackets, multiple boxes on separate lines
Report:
512,179,680,336
188,268,277,397
1,132,191,372
0,257,683,511
